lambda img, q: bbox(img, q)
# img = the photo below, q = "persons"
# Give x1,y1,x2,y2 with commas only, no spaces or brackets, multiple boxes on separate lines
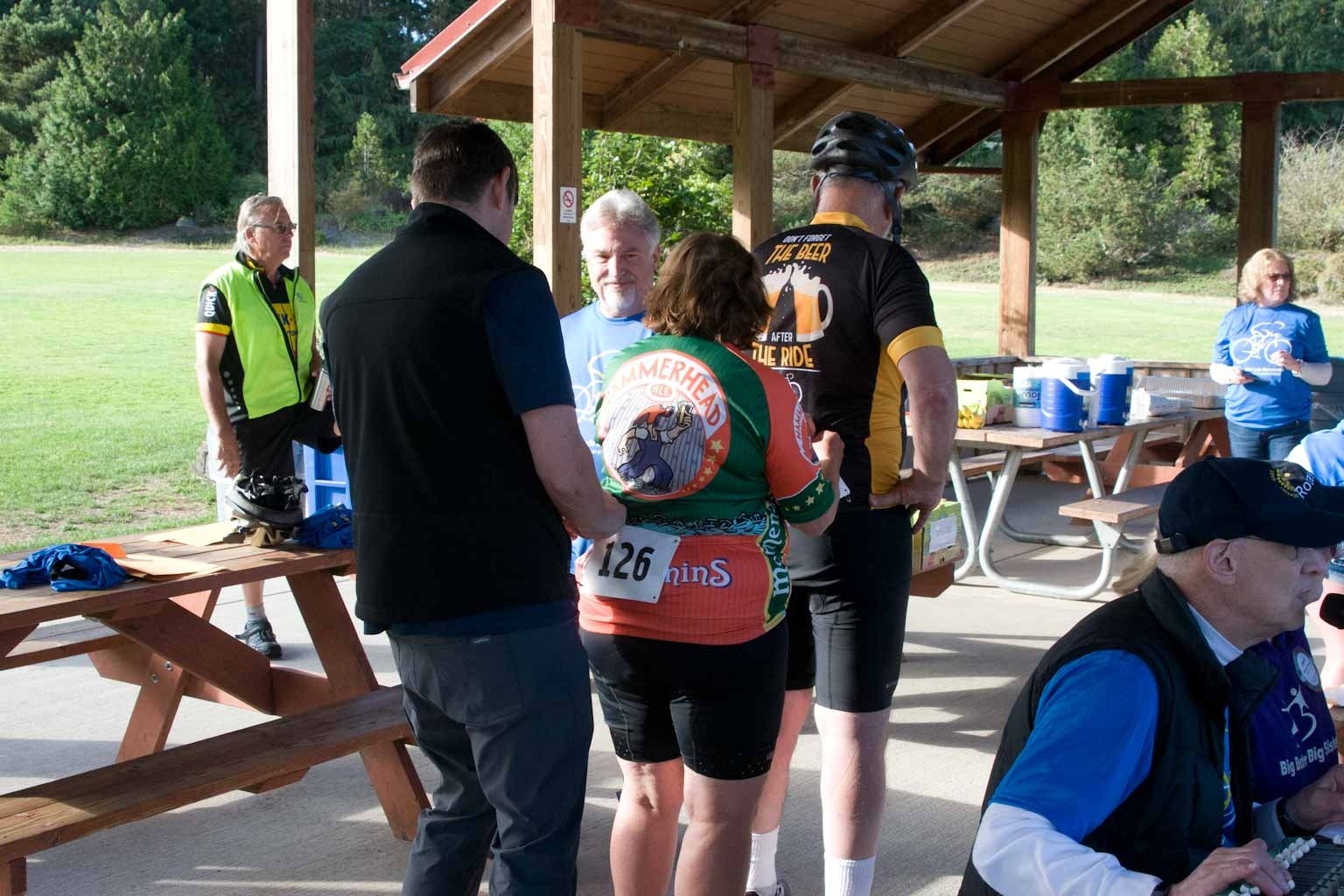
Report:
318,118,627,895
195,194,333,660
1246,460,1343,800
956,452,1344,895
560,184,664,804
1209,246,1332,459
576,230,845,895
1284,420,1344,486
742,110,959,895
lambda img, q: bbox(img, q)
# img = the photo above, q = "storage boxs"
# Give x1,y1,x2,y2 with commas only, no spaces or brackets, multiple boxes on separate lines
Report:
911,499,962,574
956,372,1014,430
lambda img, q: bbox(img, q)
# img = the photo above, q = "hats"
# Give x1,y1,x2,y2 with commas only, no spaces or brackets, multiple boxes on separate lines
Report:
1153,457,1344,555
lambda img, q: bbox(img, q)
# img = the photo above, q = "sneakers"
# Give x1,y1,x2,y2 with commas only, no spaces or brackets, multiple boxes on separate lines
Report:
234,617,282,658
744,879,791,896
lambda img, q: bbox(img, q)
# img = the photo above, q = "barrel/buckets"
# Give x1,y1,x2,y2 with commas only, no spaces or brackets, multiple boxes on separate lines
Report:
1040,356,1098,433
1012,365,1041,428
1085,353,1135,427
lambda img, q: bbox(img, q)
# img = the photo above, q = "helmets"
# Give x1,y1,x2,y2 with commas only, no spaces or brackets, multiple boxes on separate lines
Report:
224,467,308,529
810,110,917,182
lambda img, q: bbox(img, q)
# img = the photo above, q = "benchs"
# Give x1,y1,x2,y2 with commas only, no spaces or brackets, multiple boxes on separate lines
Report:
1,684,412,865
897,449,1055,582
1059,481,1172,600
1048,432,1181,462
0,621,131,670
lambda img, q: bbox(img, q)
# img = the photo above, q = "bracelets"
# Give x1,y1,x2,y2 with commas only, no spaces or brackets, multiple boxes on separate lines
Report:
1273,797,1319,836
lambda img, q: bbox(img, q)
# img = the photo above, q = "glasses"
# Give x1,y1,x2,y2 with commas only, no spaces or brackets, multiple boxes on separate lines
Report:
252,223,298,234
1267,273,1293,282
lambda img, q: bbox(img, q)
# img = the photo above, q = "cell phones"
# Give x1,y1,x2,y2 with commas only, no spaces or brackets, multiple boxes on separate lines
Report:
1240,371,1258,380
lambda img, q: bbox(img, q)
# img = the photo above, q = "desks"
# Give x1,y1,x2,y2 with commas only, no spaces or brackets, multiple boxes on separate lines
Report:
907,413,1184,601
1070,407,1231,526
0,536,430,841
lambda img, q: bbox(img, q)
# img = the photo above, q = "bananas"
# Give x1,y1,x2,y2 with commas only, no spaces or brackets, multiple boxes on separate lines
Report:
956,405,986,429
